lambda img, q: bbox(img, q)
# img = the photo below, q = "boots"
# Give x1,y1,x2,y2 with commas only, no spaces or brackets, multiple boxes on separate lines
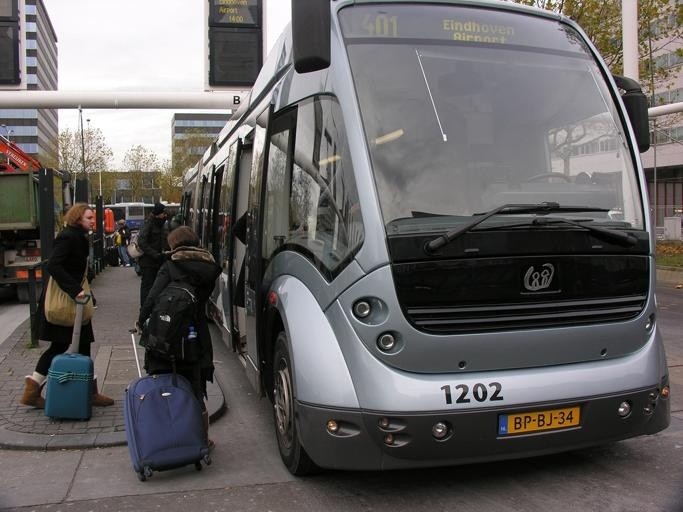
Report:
20,374,47,409
94,375,114,406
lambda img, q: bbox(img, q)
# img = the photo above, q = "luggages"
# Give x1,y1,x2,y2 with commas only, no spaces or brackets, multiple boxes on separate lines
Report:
123,328,212,482
44,294,94,421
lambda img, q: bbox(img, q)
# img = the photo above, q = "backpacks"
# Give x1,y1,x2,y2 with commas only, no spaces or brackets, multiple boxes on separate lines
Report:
139,260,217,370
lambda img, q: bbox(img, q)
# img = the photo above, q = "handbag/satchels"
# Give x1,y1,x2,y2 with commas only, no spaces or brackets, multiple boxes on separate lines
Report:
128,229,144,259
45,275,94,327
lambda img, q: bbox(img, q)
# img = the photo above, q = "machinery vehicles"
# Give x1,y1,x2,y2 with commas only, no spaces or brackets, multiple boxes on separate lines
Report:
0,131,73,308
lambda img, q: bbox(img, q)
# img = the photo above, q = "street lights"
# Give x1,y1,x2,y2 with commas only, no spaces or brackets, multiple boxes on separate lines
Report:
86,117,91,173
0,123,15,170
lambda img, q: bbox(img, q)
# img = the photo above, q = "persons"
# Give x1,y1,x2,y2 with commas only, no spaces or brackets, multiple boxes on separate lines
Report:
20,201,115,409
136,202,171,323
231,208,248,307
169,214,184,230
128,226,225,448
115,219,132,267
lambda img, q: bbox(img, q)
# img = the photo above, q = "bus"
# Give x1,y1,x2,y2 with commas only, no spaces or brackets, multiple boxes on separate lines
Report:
185,2,671,477
95,201,161,233
